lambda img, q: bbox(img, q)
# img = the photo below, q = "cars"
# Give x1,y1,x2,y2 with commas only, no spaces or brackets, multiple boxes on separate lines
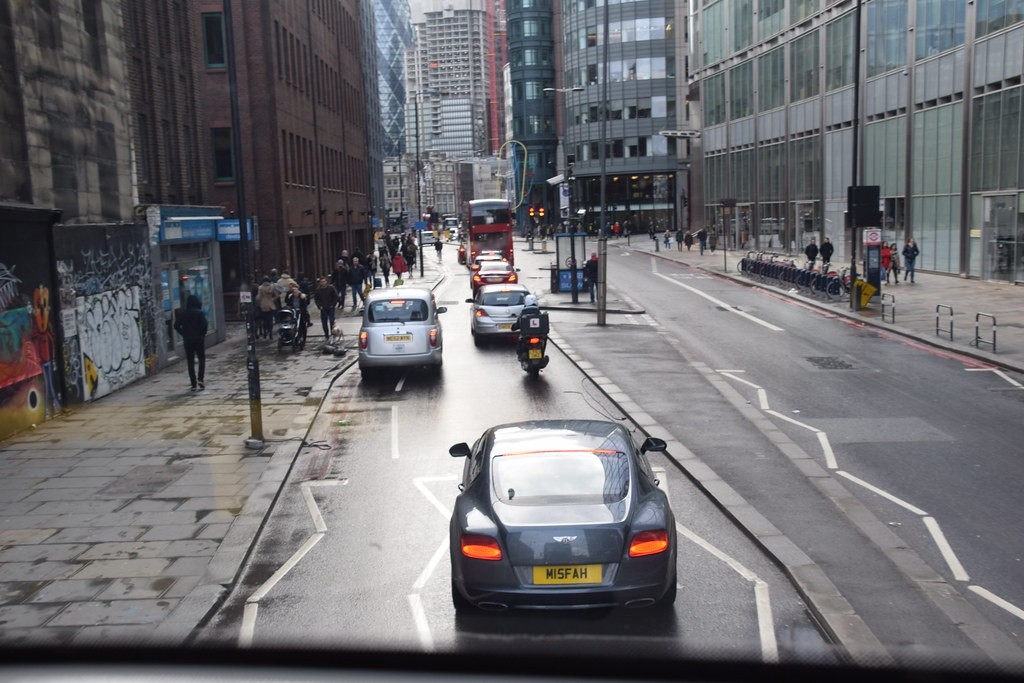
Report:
470,255,502,287
458,244,466,264
418,231,435,245
448,419,677,618
466,283,531,345
472,261,520,298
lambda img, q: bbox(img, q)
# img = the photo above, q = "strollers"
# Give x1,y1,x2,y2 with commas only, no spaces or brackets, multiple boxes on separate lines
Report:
277,306,303,350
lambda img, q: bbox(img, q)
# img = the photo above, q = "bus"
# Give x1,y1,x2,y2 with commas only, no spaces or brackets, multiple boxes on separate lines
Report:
465,200,514,268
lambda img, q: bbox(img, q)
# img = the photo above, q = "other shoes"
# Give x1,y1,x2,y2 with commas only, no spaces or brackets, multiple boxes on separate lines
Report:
895,279,899,284
191,386,196,391
887,279,889,282
269,335,272,339
307,323,313,327
362,297,368,305
264,334,267,338
352,304,358,308
904,277,907,280
911,279,915,283
198,381,205,389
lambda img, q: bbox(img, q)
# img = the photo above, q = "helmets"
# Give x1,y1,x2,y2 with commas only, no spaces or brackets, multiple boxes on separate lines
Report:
525,294,538,306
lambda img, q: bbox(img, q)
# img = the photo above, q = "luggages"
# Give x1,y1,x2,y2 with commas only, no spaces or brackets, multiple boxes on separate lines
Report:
375,277,382,287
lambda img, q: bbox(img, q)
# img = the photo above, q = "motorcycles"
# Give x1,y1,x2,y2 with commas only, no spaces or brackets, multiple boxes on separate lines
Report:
510,313,550,378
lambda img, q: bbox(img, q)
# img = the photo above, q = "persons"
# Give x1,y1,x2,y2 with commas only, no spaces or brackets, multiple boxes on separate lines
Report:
684,232,693,250
648,222,654,238
534,220,630,240
819,239,833,263
805,240,818,269
676,228,683,251
434,239,442,257
697,228,707,255
664,229,671,249
511,295,542,360
173,295,208,391
902,238,919,282
709,228,718,253
880,241,899,284
314,276,338,340
585,252,598,303
334,235,417,311
252,269,313,339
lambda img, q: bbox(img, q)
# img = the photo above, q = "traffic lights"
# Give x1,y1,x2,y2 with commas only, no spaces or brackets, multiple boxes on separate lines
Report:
539,208,544,216
530,207,534,216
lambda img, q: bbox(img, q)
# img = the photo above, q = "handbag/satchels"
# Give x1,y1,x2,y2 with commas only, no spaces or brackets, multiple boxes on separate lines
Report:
668,237,673,244
363,284,371,297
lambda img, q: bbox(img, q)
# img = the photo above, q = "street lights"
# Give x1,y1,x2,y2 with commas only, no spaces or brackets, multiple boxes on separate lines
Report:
544,87,585,302
415,89,439,277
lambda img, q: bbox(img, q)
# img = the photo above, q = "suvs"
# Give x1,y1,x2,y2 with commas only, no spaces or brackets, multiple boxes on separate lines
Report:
358,288,447,371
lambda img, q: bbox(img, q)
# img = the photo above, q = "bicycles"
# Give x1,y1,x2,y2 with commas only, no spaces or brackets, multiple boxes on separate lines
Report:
826,266,863,298
737,249,789,286
810,261,838,294
779,257,812,284
